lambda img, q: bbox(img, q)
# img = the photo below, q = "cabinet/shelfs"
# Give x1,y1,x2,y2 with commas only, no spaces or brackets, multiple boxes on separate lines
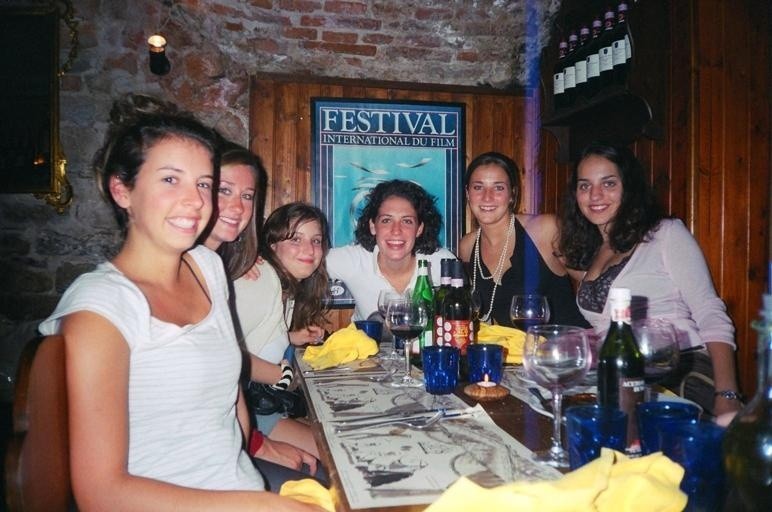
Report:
720,291,771,511
599,288,643,453
551,3,634,111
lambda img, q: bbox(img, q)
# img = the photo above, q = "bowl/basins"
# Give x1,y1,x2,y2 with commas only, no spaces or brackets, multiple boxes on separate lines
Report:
470,212,515,321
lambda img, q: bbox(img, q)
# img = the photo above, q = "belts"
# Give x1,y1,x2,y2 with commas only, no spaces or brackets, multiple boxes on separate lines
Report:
420,344,459,413
373,258,476,388
521,323,588,468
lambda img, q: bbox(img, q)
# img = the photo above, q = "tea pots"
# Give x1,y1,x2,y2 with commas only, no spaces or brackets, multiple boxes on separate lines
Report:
0,0,81,214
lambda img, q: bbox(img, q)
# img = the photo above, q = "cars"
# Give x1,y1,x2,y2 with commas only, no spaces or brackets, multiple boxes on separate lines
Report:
566,405,627,472
467,345,503,384
511,296,549,329
636,316,681,385
633,399,705,498
355,319,382,348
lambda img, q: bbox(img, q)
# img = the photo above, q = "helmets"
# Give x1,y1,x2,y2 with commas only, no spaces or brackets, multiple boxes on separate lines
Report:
529,386,674,423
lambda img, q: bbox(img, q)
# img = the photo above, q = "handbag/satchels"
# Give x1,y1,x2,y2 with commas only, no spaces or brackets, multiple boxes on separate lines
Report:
269,358,293,392
247,429,264,456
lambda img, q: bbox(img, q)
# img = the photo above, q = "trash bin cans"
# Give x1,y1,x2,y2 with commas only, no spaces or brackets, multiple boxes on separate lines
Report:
714,390,744,404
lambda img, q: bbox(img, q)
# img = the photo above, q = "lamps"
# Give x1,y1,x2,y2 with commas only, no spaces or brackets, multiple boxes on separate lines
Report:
2,334,77,512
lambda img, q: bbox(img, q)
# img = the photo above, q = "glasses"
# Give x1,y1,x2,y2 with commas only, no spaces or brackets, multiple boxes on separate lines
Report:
148,36,170,74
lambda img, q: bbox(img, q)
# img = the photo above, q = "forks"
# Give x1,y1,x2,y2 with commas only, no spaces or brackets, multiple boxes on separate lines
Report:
337,410,442,436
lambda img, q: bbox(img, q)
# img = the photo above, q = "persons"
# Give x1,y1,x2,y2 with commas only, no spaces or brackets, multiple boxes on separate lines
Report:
552,143,744,426
239,180,459,322
39,94,334,511
459,153,579,336
225,203,322,462
201,140,330,492
